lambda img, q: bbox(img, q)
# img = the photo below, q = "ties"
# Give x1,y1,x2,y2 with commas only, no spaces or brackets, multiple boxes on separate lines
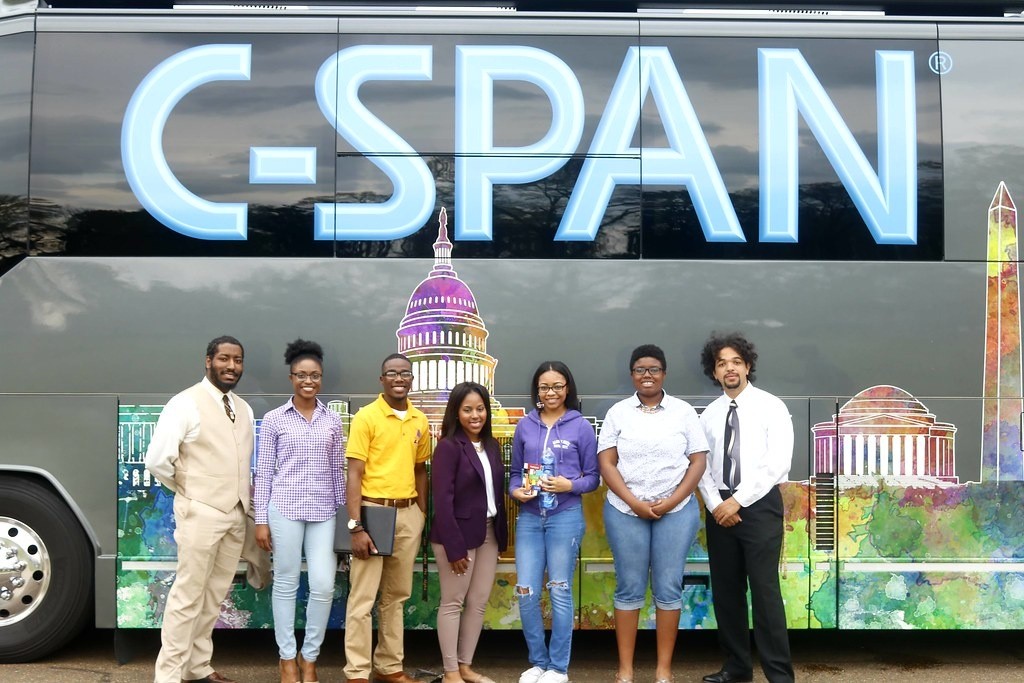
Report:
223,395,235,423
722,400,741,491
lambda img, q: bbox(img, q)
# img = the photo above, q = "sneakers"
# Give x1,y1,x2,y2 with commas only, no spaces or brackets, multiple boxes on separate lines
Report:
536,671,568,683
519,666,545,683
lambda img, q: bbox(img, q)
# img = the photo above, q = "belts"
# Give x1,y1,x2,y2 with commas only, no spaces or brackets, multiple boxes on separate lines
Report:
361,495,416,508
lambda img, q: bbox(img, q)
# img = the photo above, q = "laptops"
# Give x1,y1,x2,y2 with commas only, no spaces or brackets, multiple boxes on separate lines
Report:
334,506,398,556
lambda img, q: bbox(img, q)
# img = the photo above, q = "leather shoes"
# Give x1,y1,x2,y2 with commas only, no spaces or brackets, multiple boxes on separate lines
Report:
182,671,237,683
373,671,424,683
347,677,369,683
702,669,749,683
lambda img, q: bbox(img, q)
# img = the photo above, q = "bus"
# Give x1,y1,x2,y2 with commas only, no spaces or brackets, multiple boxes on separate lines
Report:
1,1,1023,663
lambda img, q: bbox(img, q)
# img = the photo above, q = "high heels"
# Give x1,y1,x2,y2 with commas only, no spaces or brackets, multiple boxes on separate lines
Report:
279,658,301,683
296,651,319,683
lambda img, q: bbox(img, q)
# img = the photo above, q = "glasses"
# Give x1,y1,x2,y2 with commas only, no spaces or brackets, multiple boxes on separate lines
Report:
292,372,322,382
537,383,567,393
383,371,412,380
632,367,664,375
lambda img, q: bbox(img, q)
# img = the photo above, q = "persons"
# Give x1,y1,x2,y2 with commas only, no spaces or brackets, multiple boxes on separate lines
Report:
426,381,508,682
254,337,346,683
699,329,799,683
594,344,712,683
342,354,432,683
143,336,257,683
505,361,601,683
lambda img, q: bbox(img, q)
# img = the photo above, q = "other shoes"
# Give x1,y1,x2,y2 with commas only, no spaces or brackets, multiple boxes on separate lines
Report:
473,675,495,683
443,677,465,683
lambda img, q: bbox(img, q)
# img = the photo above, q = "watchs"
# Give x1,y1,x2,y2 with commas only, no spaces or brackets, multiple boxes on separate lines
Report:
348,518,364,530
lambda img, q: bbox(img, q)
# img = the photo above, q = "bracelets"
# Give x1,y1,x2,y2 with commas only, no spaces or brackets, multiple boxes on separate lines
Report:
350,528,364,534
